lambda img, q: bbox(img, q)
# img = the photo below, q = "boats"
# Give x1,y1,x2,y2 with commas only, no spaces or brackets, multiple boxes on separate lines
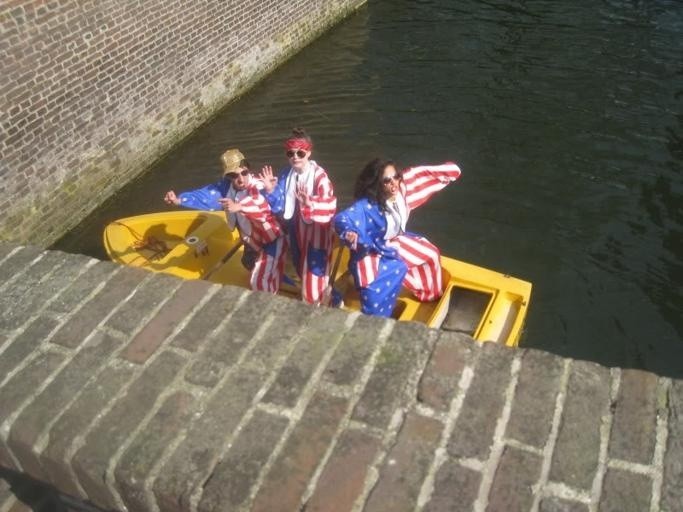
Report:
102,209,533,347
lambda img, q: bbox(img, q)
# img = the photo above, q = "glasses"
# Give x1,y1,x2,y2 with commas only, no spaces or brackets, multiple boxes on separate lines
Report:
384,173,400,183
286,150,306,158
230,170,247,178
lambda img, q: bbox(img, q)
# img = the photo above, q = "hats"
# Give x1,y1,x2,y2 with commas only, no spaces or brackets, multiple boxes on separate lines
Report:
220,149,244,178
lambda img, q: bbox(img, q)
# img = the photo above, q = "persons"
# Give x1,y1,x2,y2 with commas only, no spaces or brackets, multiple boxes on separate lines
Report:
334,158,461,317
164,149,291,294
258,126,344,308
